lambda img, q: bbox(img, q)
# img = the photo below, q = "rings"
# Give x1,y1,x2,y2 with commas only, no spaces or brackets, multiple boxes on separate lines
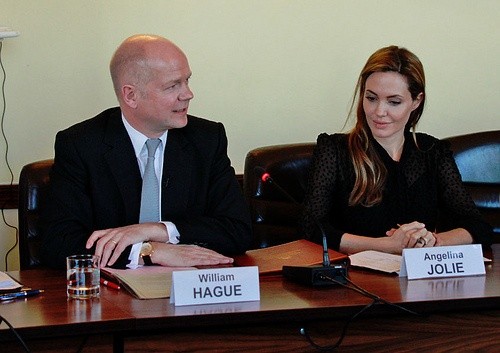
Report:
420,237,426,247
110,239,116,244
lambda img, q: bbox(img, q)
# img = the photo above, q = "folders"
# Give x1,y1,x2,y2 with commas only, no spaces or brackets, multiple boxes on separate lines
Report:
234,239,353,281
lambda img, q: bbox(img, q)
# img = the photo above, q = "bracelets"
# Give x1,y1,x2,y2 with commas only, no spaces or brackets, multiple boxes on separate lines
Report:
432,233,438,247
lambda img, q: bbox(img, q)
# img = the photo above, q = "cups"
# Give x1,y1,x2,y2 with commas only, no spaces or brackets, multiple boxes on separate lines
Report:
66,254,101,300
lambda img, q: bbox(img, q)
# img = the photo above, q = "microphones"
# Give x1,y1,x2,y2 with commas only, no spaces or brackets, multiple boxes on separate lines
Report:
261,173,349,287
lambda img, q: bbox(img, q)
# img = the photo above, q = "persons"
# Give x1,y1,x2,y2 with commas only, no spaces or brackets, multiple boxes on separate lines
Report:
35,34,257,268
302,46,496,253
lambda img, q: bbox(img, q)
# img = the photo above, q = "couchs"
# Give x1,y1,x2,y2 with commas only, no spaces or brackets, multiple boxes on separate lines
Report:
245,143,319,250
443,130,500,244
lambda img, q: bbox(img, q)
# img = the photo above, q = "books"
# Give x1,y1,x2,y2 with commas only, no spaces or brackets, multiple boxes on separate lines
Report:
237,239,350,274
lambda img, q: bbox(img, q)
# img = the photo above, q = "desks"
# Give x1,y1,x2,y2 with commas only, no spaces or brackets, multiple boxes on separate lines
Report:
0,255,500,353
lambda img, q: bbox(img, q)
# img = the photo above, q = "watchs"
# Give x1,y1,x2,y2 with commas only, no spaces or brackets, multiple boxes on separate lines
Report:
140,240,153,265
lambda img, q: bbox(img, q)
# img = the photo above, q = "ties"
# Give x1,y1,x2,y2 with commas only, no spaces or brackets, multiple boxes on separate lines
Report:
139,138,160,224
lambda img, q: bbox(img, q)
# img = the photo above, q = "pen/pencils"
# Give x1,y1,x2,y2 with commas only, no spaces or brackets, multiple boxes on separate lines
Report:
1,288,48,302
397,223,424,246
100,278,120,290
